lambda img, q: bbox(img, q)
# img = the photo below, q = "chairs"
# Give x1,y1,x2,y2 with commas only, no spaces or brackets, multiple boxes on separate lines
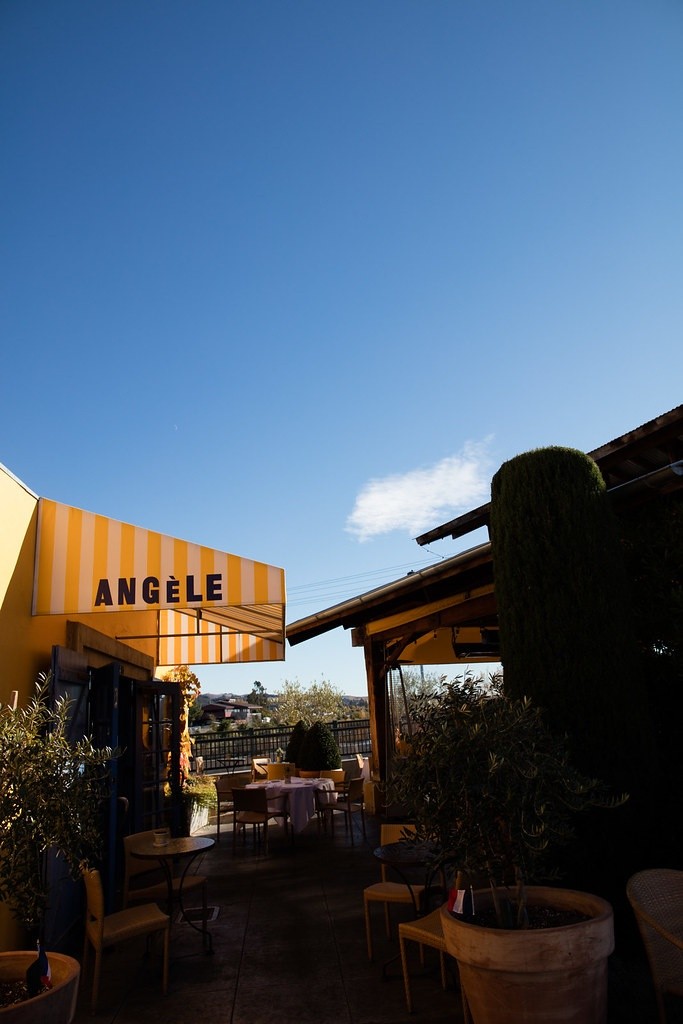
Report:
363,824,438,965
252,758,272,782
79,859,170,1016
124,826,208,954
626,867,683,1024
213,773,253,841
231,788,290,854
398,867,472,1024
314,777,365,844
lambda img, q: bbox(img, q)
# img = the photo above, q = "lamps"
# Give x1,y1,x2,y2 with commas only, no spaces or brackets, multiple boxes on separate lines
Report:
449,625,502,659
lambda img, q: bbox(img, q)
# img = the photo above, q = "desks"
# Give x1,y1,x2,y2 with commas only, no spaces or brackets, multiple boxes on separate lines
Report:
216,759,245,775
236,778,338,838
373,840,445,921
131,836,216,964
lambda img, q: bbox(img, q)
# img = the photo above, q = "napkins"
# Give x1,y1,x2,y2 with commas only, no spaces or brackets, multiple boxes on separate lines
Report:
303,781,314,785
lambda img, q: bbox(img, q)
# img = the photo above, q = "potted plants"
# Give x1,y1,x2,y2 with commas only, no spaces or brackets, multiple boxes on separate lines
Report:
372,677,630,1024
275,747,286,762
0,670,128,1024
299,720,346,785
284,720,310,778
164,775,219,834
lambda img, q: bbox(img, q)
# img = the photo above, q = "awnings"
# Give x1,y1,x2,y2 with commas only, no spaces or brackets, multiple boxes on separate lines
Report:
31,497,287,667
